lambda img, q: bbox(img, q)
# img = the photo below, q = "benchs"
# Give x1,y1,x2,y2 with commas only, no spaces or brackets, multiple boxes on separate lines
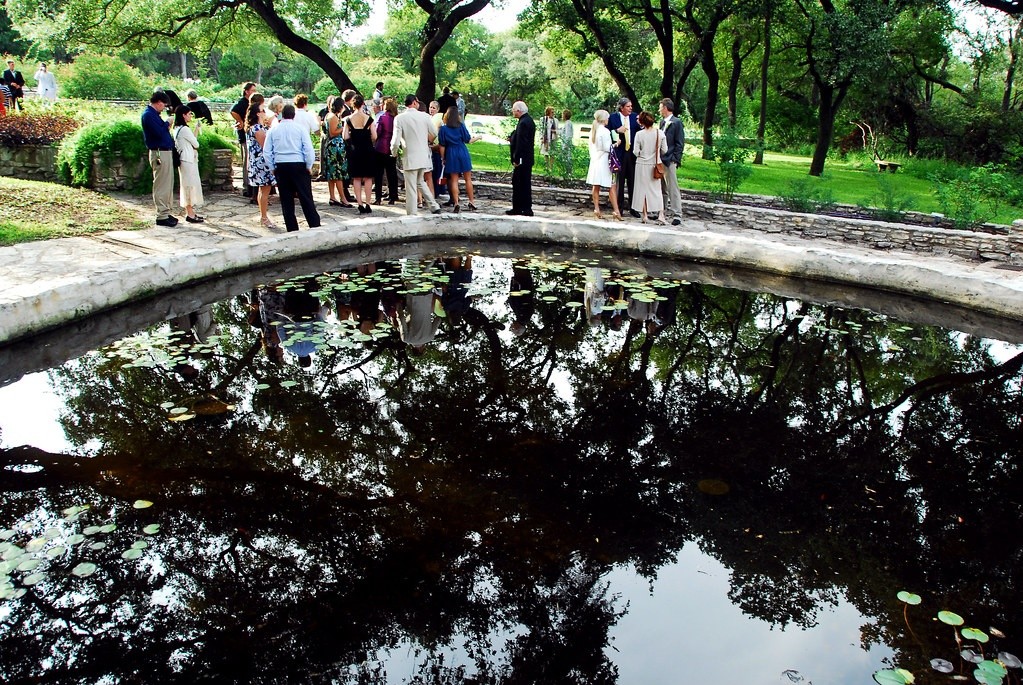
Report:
875,160,902,173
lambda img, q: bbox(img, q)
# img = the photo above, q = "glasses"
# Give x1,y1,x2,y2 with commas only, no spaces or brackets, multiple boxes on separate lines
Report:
258,109,266,114
416,101,420,104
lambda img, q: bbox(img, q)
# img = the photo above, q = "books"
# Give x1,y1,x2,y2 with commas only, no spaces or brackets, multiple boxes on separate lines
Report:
513,158,522,166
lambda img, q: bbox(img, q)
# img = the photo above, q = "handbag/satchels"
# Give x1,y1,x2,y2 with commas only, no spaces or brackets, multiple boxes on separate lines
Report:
653,163,665,180
608,144,622,172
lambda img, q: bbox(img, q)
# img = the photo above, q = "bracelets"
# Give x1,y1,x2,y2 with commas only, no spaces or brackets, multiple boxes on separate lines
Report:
441,158,444,160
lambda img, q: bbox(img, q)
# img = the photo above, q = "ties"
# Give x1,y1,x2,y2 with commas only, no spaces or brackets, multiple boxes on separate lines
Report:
624,116,630,152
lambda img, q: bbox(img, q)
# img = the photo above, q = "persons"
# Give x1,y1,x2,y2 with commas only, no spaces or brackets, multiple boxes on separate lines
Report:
33,62,56,102
243,103,276,229
229,82,483,215
56,58,60,65
141,86,213,227
0,61,25,117
170,257,679,379
586,98,684,226
540,107,573,177
3,51,7,59
615,143,618,147
505,101,535,216
193,75,202,84
263,105,321,232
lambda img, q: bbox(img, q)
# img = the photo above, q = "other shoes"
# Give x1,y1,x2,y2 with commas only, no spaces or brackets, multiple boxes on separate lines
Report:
382,192,389,200
329,198,354,208
186,214,204,223
156,215,179,227
348,196,357,202
671,220,680,225
630,209,641,218
648,215,658,220
505,209,534,217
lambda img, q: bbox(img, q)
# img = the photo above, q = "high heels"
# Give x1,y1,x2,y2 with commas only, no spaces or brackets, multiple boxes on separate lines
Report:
454,205,461,214
612,212,625,221
260,216,275,228
443,200,455,208
467,202,478,211
594,210,605,220
372,198,395,205
658,217,667,226
357,204,372,215
642,217,648,224
